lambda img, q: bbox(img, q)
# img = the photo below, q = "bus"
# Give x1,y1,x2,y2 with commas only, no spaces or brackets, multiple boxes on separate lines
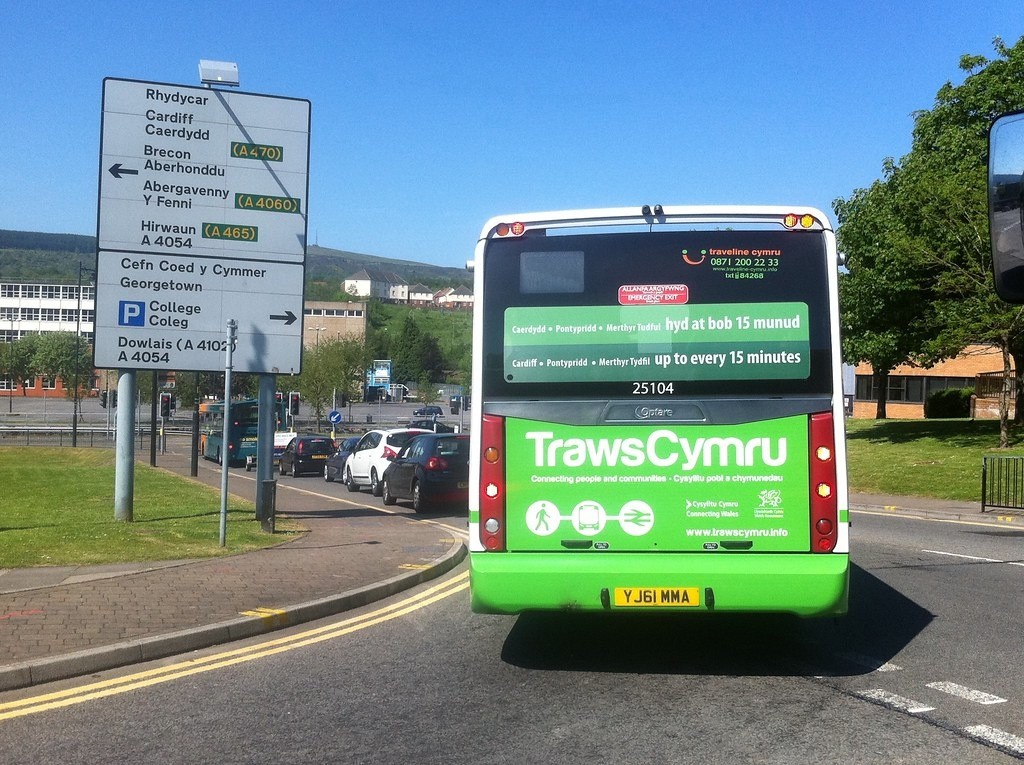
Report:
467,202,853,612
197,400,286,465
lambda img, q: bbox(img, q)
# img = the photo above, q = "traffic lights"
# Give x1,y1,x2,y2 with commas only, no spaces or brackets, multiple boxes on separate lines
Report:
276,392,282,403
159,392,175,417
288,392,301,416
449,394,470,415
110,390,117,408
100,390,107,408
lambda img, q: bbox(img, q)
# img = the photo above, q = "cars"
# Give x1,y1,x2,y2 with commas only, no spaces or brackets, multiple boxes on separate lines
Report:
405,420,455,435
321,441,359,483
379,433,475,518
278,435,332,475
413,406,443,417
342,428,437,498
246,432,297,472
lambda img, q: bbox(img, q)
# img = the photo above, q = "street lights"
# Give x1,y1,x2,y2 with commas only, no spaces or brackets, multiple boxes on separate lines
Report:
308,325,327,347
